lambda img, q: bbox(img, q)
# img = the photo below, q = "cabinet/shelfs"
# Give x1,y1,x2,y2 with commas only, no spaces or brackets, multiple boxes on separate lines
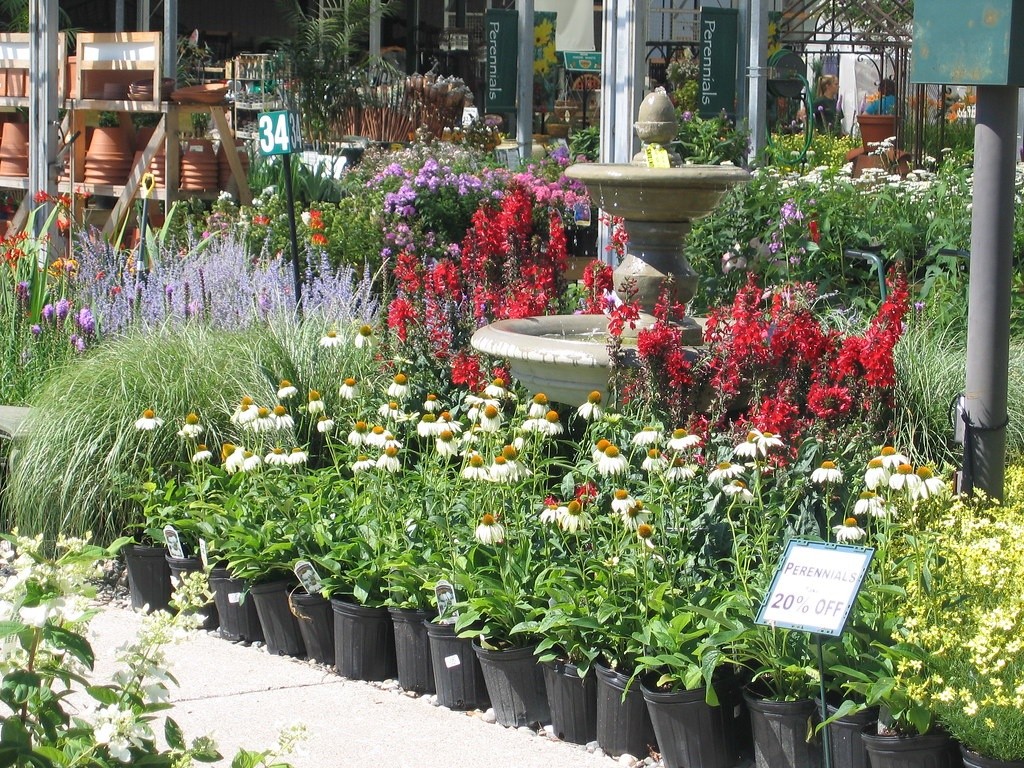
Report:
234,54,298,140
0,32,258,243
181,58,231,139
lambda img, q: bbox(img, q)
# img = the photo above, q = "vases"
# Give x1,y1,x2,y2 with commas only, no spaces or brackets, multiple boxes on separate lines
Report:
0,56,250,269
124,543,1024,768
855,115,901,155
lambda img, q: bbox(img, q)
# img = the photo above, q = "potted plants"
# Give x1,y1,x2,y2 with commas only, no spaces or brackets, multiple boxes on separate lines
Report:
546,99,599,136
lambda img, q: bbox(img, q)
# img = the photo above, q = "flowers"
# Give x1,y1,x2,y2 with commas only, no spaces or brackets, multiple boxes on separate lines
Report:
122,323,954,743
882,484,1024,759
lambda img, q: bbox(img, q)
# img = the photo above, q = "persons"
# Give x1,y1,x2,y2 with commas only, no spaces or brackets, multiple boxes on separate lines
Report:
862,78,895,115
800,75,846,138
